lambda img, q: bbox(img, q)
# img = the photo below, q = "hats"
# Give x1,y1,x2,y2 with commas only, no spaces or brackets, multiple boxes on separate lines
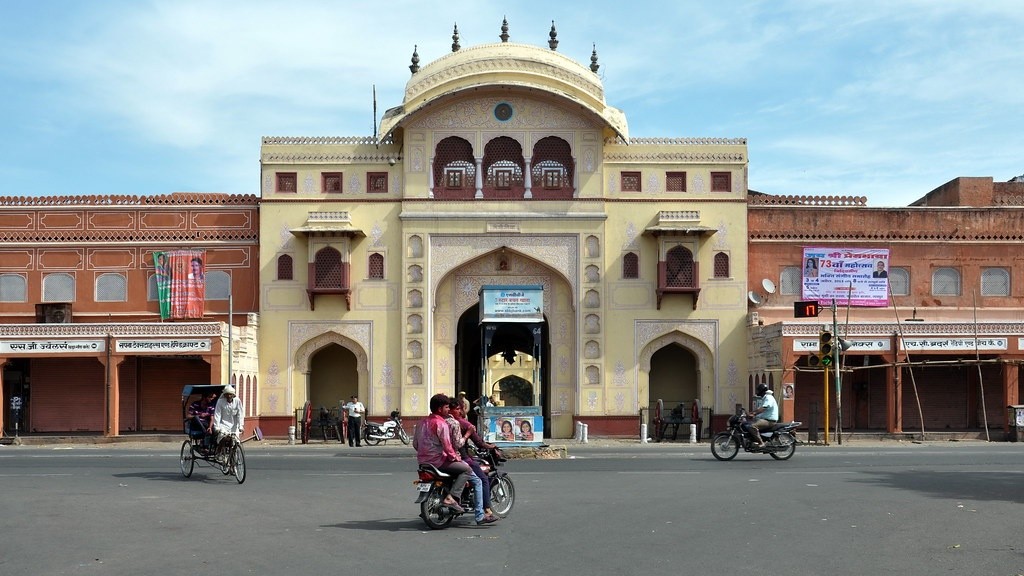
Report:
458,391,466,395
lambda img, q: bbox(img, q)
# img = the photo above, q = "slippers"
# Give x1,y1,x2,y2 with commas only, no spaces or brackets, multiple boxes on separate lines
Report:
442,500,465,512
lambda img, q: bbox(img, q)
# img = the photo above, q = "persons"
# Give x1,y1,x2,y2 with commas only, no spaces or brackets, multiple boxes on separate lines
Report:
188,257,204,279
156,254,172,282
498,418,514,441
186,390,215,453
456,402,500,520
804,258,818,277
413,393,472,512
213,385,245,476
342,394,365,447
459,391,470,422
873,261,887,278
784,385,793,399
748,384,779,448
443,396,497,526
473,395,497,407
515,420,533,442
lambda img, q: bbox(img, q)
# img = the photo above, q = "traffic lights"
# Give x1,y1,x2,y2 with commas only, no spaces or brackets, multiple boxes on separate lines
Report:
793,300,819,318
807,330,833,367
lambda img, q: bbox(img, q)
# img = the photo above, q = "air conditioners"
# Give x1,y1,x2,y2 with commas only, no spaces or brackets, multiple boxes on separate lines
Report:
45,309,71,323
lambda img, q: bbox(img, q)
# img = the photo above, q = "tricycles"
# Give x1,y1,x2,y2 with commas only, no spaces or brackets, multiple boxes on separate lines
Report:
179,384,247,485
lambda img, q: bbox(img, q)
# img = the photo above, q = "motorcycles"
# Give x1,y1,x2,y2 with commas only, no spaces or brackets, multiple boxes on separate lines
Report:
710,407,804,462
362,408,410,446
413,442,517,530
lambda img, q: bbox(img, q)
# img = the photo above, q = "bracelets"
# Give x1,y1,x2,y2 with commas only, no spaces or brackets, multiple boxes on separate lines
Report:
464,435,468,439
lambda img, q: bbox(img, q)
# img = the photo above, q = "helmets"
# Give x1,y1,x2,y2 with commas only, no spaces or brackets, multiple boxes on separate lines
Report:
756,383,768,396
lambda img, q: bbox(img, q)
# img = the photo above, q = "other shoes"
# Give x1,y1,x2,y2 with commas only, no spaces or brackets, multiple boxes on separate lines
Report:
350,443,353,447
758,442,765,447
477,514,493,525
356,444,361,447
485,512,500,522
